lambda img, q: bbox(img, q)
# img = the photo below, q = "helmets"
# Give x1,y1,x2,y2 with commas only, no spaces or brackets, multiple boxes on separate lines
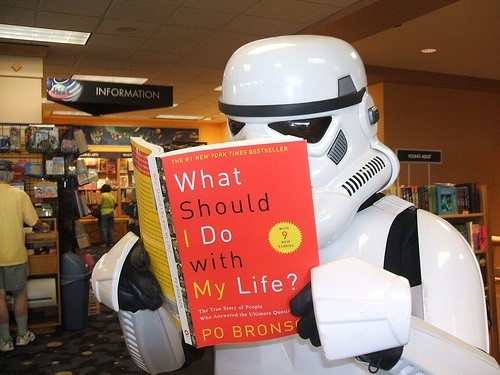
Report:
218,34,400,251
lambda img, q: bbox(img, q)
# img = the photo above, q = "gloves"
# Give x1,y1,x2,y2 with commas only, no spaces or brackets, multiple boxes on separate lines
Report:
288,280,403,373
117,240,163,314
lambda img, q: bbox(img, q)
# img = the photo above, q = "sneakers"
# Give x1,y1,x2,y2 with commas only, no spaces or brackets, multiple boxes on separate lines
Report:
0,336,14,352
16,330,35,345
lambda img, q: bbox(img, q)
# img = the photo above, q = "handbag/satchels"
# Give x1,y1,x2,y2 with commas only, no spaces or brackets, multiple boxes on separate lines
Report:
92,208,101,218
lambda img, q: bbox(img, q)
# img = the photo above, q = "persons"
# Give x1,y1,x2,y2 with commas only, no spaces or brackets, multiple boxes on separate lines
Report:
0,160,42,351
97,184,116,248
91,35,500,375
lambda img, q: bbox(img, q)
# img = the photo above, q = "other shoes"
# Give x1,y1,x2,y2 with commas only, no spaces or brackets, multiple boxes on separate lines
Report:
109,243,113,247
99,244,106,248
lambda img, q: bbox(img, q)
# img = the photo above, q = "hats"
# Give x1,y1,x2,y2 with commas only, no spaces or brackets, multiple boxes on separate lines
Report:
0,160,13,171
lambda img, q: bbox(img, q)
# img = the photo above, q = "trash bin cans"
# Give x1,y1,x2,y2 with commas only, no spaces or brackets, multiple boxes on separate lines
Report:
61,251,91,329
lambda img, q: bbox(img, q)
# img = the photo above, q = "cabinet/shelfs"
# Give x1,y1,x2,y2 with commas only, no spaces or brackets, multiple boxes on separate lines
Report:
436,185,500,365
0,122,135,335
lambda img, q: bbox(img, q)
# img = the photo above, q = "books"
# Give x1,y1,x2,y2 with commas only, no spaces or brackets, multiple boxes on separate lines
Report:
129,136,320,349
401,183,488,251
